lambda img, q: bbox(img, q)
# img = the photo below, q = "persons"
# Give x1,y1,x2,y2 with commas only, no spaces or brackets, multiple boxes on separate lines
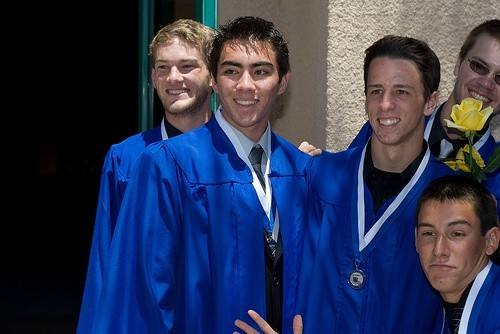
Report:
77,19,218,334
89,15,314,334
306,35,462,334
297,19,500,207
413,175,500,334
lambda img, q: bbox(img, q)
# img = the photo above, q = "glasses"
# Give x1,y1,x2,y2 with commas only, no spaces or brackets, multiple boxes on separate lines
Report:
463,55,500,85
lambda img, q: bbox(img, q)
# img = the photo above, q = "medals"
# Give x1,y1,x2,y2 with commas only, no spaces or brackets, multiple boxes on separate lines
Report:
348,269,366,290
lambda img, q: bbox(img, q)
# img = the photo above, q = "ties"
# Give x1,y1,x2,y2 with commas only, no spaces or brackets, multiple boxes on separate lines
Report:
248,147,268,194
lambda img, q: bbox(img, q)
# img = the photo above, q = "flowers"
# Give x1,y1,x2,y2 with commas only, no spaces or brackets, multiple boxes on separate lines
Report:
433,98,500,183
